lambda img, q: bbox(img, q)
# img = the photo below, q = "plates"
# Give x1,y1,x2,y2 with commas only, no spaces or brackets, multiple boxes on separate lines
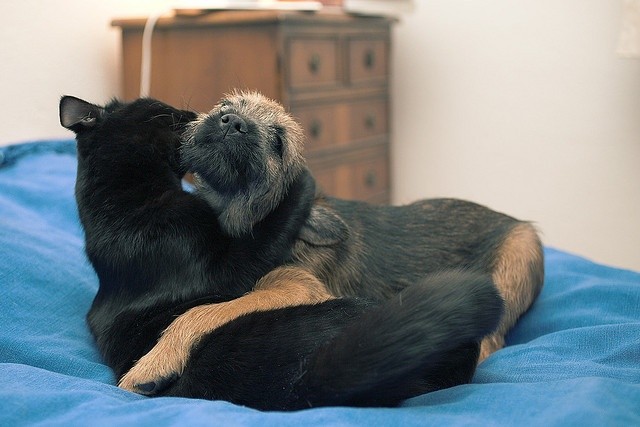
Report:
110,10,399,202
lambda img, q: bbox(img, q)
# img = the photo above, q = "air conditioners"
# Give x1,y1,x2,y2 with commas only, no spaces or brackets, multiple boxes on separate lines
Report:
59,95,506,411
117,87,545,397
